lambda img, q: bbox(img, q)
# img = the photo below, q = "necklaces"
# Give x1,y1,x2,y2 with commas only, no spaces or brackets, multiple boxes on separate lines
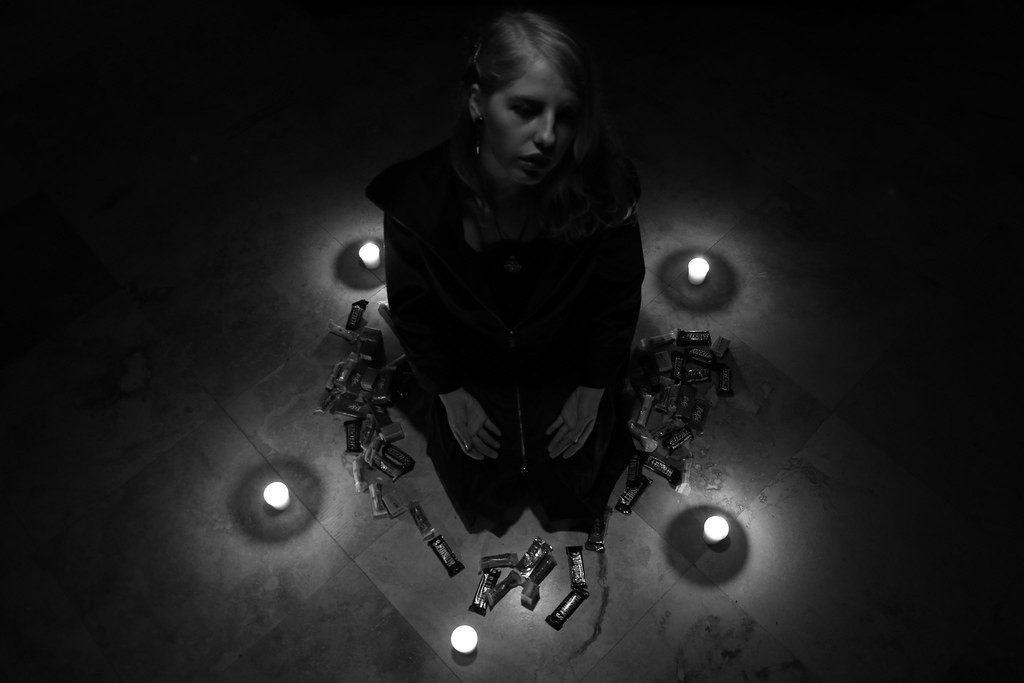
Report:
489,193,535,277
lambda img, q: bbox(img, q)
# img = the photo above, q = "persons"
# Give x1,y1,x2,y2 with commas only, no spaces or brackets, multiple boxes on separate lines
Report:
366,12,648,540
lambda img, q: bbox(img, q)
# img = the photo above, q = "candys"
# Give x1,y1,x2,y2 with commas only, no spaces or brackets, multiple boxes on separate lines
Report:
314,298,733,629
